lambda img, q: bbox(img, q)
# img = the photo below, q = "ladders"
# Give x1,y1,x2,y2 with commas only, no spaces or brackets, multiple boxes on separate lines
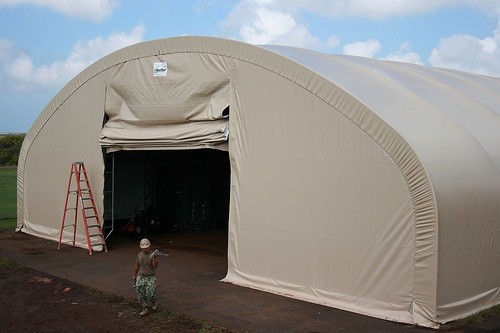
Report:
57,162,108,256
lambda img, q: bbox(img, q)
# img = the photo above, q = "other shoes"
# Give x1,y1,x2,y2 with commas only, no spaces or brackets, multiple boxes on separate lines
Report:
152,303,157,310
140,308,148,315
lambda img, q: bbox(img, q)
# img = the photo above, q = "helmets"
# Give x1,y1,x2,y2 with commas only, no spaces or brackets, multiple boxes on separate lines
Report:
140,239,151,248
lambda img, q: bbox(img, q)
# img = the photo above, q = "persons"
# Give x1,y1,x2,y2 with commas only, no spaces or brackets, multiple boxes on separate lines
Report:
133,238,160,316
